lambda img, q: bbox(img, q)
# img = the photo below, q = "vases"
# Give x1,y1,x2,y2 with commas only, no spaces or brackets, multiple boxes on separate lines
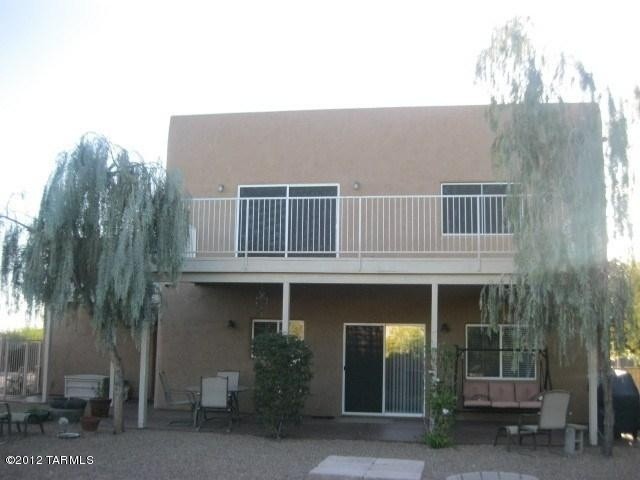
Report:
80,417,101,431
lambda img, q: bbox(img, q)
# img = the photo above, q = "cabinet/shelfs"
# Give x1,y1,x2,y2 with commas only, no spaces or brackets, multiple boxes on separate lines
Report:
64,374,109,402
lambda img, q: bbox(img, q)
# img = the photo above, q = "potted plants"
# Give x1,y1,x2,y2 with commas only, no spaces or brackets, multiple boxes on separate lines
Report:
87,380,112,417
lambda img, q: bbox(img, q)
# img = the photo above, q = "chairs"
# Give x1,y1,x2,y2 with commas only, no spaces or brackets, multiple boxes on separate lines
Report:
1,399,13,439
522,389,572,454
158,368,269,433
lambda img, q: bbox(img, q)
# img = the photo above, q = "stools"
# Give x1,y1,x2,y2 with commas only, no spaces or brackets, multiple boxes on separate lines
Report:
494,425,537,453
10,411,45,437
